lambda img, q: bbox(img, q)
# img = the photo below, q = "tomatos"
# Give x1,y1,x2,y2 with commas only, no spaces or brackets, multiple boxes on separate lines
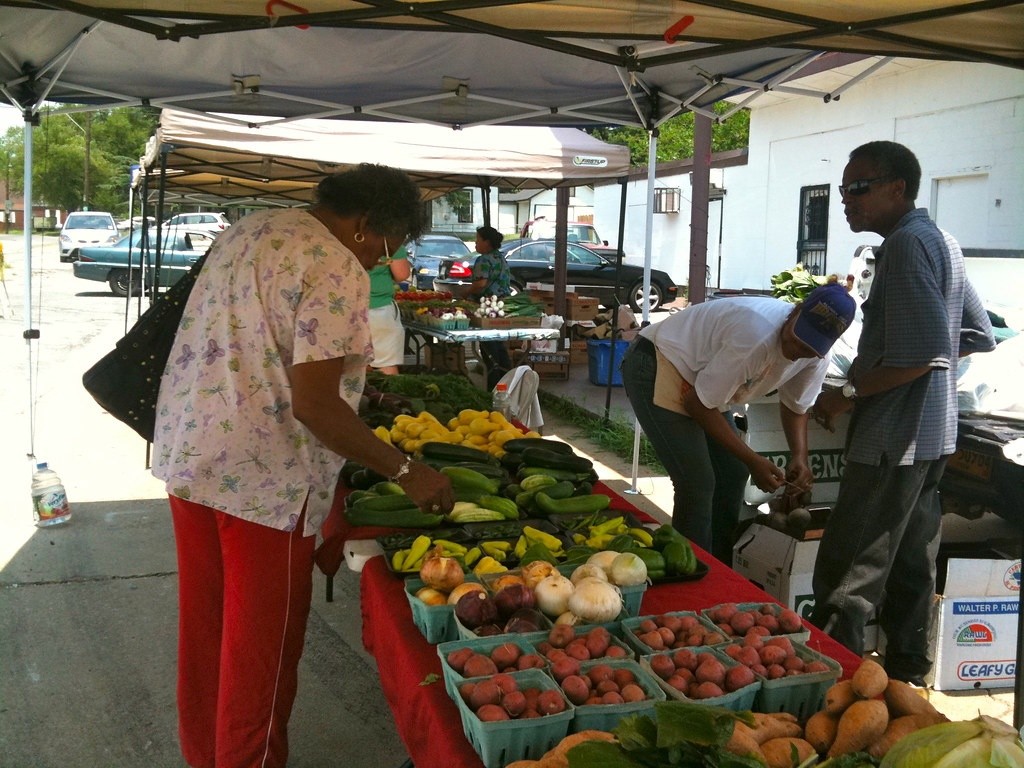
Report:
395,290,452,301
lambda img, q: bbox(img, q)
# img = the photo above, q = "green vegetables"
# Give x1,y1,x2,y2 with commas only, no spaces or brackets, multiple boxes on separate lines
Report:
358,370,497,428
564,697,1023,768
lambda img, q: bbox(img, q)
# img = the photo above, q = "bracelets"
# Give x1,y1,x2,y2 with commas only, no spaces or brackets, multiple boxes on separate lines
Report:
391,455,413,484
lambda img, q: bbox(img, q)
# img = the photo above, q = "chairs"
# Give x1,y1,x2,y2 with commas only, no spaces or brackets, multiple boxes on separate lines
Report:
491,365,545,436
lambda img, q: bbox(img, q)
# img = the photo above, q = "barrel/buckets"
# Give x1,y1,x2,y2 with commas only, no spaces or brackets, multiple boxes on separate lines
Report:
587,339,630,387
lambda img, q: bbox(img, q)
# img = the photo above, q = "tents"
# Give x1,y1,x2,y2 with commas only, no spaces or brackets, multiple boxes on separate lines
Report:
1,0,1024,529
124,104,630,428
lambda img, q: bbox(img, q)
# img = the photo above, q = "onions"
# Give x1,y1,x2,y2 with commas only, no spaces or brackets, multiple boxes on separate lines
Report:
473,295,506,318
417,551,648,635
423,307,468,320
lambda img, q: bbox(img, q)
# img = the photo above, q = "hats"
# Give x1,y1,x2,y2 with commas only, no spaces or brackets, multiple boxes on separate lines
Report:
790,281,857,357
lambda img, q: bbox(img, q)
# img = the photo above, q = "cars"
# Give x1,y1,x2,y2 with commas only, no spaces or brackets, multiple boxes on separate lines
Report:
151,211,232,234
433,236,679,313
405,234,473,292
55,210,126,262
117,215,156,232
72,226,218,297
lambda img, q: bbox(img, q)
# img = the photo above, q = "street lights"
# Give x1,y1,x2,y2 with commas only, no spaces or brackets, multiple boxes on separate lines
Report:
3,149,17,234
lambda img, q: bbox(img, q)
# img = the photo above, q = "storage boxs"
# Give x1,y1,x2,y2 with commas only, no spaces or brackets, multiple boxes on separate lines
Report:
735,507,879,651
421,282,644,386
404,563,844,768
932,541,1023,692
733,402,851,506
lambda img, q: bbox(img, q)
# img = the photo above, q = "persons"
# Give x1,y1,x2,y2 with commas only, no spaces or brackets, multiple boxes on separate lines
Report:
461,227,512,390
149,161,456,768
810,140,996,692
618,283,857,570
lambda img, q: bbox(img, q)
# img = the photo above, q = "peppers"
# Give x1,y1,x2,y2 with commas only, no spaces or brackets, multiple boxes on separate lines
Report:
394,508,698,579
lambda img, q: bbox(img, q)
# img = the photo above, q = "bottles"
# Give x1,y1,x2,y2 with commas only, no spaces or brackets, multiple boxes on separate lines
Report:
32,461,72,528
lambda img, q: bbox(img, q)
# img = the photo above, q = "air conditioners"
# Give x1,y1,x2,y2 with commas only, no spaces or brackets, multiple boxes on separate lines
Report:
654,188,681,213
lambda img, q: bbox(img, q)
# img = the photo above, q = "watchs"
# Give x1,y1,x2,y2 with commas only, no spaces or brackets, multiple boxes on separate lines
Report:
842,383,857,399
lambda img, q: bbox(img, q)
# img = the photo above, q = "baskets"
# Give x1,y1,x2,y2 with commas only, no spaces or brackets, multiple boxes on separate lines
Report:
406,565,845,768
403,307,470,331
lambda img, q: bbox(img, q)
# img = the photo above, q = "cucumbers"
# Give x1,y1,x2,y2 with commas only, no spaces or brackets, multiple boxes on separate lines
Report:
339,437,615,528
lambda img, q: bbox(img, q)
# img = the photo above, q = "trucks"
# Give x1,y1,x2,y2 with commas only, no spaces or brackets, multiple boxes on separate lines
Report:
841,242,1024,534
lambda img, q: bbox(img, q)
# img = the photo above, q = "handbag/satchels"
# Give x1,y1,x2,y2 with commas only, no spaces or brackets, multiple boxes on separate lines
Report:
83,239,218,443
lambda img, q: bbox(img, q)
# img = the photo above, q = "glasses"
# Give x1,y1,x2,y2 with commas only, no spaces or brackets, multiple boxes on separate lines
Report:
373,236,393,266
838,173,897,197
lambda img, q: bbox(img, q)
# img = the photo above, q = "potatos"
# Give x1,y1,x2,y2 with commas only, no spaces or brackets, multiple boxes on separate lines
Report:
448,603,956,768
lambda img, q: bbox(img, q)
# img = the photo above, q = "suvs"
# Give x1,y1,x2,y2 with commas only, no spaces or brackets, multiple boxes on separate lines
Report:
520,218,626,264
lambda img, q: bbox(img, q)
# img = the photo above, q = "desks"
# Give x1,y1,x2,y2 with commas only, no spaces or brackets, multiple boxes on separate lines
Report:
399,318,560,386
313,475,886,768
